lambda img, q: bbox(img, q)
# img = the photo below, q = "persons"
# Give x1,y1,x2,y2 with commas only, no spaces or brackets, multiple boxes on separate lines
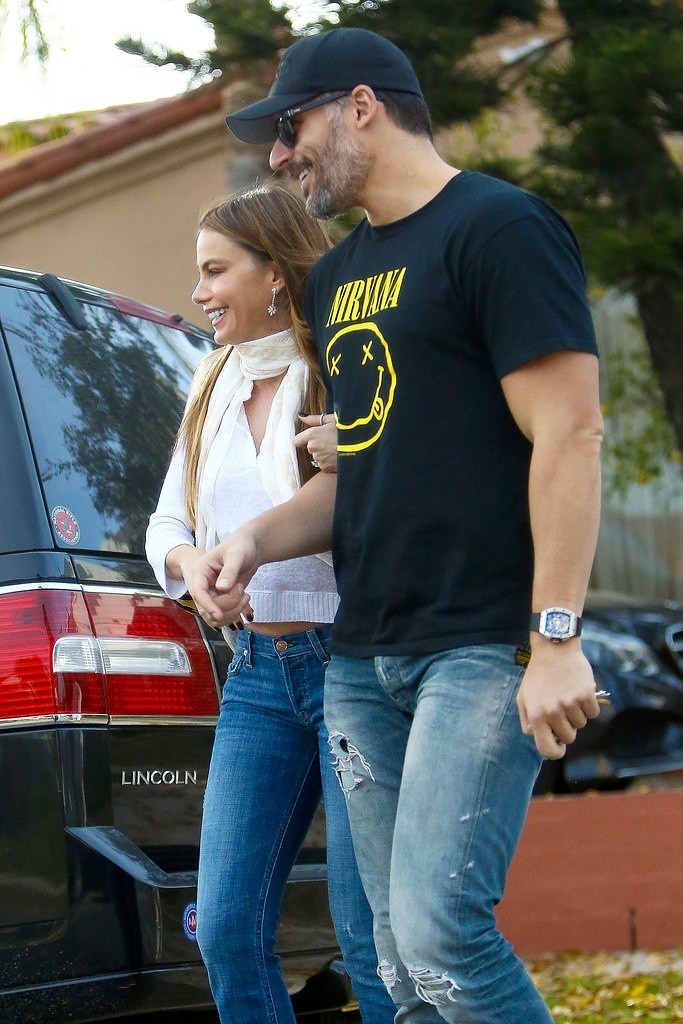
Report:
189,29,612,1024
145,182,398,1024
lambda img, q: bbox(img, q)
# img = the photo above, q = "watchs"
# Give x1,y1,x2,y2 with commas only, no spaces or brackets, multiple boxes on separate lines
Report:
528,607,581,645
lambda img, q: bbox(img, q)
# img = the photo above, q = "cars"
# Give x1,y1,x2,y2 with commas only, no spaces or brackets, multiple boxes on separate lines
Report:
2,259,366,1024
527,585,682,795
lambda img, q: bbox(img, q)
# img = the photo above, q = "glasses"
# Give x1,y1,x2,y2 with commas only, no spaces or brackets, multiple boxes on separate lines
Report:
274,90,384,146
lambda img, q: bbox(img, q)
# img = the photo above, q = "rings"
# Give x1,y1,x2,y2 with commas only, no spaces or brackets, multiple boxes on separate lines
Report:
312,461,319,468
319,413,326,427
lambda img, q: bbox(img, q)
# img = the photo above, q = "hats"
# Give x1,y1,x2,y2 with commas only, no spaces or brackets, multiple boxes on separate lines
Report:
225,27,421,146
